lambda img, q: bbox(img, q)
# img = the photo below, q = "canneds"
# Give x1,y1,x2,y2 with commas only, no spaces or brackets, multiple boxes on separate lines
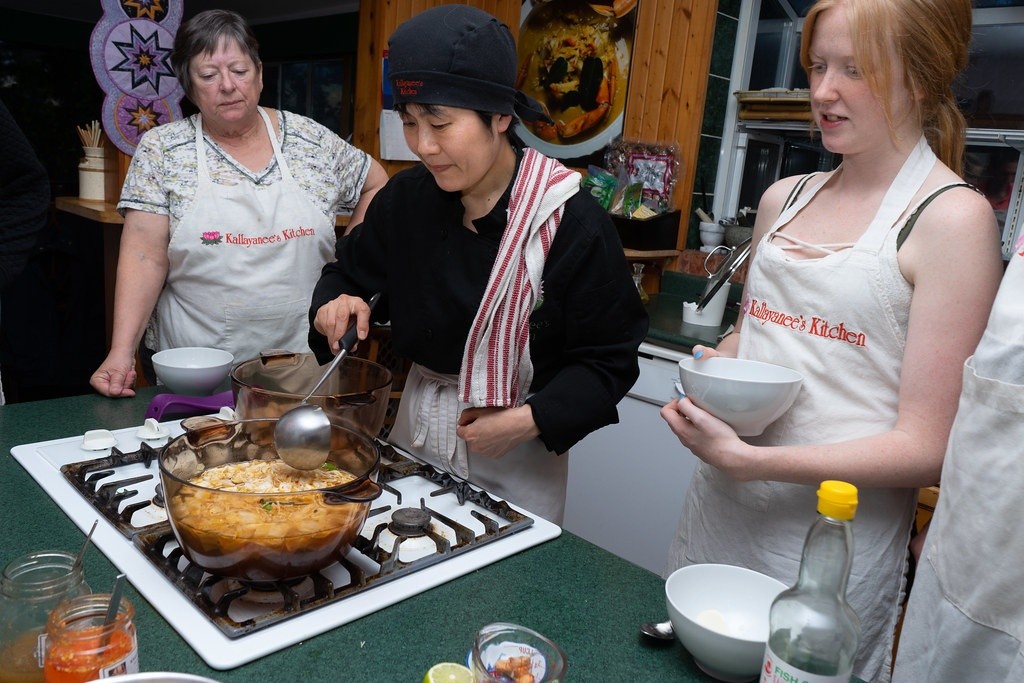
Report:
2,549,138,683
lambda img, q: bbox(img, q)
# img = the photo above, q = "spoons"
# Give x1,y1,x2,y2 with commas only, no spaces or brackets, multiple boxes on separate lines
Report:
67,518,99,573
640,617,679,642
275,290,392,471
95,571,129,657
142,389,233,424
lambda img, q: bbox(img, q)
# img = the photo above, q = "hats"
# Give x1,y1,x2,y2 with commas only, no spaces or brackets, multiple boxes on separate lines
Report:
383,5,517,110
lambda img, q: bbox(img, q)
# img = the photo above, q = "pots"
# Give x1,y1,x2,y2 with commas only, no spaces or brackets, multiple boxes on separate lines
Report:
159,414,382,583
232,349,393,440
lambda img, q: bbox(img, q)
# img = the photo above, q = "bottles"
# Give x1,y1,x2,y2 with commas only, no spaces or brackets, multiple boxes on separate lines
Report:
78,147,118,204
1,549,94,683
43,592,140,682
757,479,863,682
630,262,649,304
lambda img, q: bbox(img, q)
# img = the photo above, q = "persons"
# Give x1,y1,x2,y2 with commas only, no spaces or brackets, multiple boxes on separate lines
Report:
659,0,1004,683
88,10,390,397
889,225,1024,683
306,5,646,529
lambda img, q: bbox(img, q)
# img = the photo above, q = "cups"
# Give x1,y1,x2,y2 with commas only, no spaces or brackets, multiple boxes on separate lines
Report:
471,621,569,683
699,221,726,253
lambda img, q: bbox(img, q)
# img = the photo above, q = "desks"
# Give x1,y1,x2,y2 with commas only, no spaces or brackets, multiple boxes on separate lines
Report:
0,383,873,683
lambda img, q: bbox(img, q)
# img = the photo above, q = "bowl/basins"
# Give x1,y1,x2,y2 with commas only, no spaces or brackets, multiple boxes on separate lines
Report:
664,561,791,683
151,346,233,399
678,356,804,436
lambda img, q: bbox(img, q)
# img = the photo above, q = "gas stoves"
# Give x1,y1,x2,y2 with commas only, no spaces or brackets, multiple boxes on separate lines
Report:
10,405,564,670
